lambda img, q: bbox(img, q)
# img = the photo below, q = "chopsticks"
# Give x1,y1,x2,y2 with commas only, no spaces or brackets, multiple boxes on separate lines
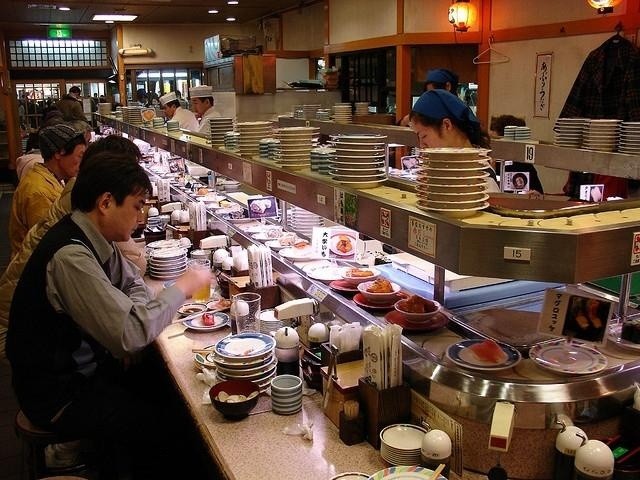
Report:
192,349,213,354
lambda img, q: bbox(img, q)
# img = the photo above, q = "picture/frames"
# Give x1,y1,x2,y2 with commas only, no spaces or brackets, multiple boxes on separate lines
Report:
532,51,554,120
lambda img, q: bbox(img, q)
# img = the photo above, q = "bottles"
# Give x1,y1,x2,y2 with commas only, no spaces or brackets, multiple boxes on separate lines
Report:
307,322,330,352
573,433,614,480
229,300,249,335
553,421,587,480
420,429,452,478
221,257,234,298
212,248,230,273
274,326,299,375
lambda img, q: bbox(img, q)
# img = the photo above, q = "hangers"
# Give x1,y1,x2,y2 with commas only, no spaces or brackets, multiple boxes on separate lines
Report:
472,36,510,65
582,24,637,65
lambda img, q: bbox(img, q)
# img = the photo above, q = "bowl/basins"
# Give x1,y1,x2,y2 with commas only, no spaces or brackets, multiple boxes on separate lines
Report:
209,379,261,422
395,299,441,324
357,279,401,303
343,267,382,280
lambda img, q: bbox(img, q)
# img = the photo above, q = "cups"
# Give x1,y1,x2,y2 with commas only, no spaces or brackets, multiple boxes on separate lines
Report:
187,259,211,303
270,375,303,416
233,292,261,333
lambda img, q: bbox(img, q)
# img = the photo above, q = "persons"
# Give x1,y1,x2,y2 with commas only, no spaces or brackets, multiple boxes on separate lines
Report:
1,136,147,361
8,126,88,260
5,154,215,433
397,68,459,128
409,89,500,196
188,85,222,138
13,64,101,138
159,92,200,134
131,85,157,107
490,114,544,194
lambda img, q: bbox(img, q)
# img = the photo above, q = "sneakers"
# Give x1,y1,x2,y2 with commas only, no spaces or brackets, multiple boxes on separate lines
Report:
42,445,91,479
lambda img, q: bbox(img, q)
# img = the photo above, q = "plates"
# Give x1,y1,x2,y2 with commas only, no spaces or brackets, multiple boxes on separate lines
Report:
148,248,188,279
327,133,389,189
529,341,607,375
224,134,227,148
330,280,359,294
244,225,284,234
386,311,448,334
307,265,350,281
320,112,329,121
316,112,320,120
153,118,164,128
273,126,320,171
183,311,229,332
213,360,277,394
214,333,276,359
380,423,427,466
303,104,320,118
581,117,621,154
206,299,231,311
256,307,282,334
330,234,356,257
291,208,325,239
515,127,532,141
234,121,274,158
177,303,207,317
252,232,296,240
554,118,581,149
319,151,332,175
167,121,179,132
287,209,292,226
194,345,214,368
265,239,309,251
445,338,522,371
504,126,517,139
268,141,279,159
618,122,639,153
310,151,319,171
452,295,561,347
353,293,412,314
205,117,234,147
332,472,371,480
415,146,492,218
330,103,353,125
260,139,274,157
608,322,640,352
367,466,447,479
354,102,369,117
227,134,239,150
278,248,312,261
128,107,143,124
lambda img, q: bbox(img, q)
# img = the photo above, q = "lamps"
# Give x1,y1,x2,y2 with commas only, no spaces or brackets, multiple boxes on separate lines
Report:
208,9,219,14
225,17,236,22
58,7,71,11
91,14,138,24
586,0,623,15
447,0,477,33
227,0,238,5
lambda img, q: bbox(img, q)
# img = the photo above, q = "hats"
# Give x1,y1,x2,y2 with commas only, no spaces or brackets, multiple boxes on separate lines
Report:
158,91,177,107
187,84,213,99
35,123,83,160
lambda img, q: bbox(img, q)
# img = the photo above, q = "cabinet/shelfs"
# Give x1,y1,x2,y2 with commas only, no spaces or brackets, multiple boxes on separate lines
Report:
275,111,640,181
202,53,264,96
91,111,640,324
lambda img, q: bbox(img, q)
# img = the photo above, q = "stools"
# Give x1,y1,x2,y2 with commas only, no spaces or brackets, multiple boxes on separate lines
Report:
14,408,86,479
